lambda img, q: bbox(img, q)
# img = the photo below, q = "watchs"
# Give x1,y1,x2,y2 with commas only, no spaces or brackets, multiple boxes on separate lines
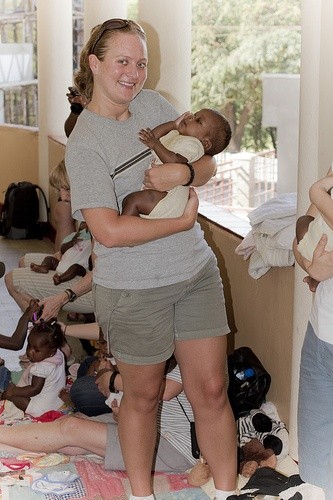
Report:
67,287,77,301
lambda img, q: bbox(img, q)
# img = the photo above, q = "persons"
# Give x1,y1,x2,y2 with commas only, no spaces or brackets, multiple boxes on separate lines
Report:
63,18,242,500
291,148,333,500
295,172,332,292
0,316,69,418
5,154,134,424
119,103,232,221
0,297,41,394
0,320,213,472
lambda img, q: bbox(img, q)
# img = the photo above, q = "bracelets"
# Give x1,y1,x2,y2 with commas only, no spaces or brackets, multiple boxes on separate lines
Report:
109,370,119,394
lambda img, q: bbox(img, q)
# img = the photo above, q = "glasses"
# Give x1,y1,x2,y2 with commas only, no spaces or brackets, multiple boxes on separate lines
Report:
91,19,144,55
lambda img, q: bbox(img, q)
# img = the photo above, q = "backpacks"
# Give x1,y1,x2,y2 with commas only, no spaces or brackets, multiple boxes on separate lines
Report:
0,182,49,240
227,347,271,422
